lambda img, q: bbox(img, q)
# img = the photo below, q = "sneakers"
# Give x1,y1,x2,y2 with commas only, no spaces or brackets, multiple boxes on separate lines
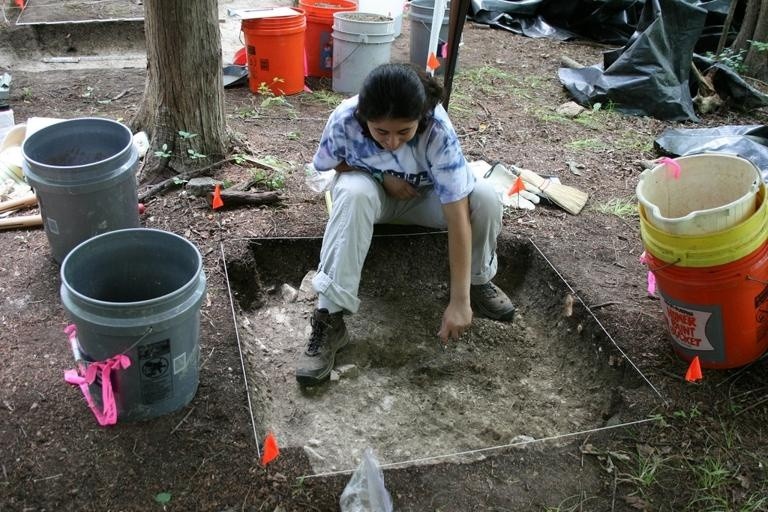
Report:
465,279,521,322
286,312,355,390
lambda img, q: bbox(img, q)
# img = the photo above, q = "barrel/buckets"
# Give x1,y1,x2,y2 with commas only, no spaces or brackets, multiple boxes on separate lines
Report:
297,1,357,76
55,227,207,421
636,154,761,237
408,1,461,77
22,117,140,266
637,184,768,371
332,11,394,96
239,6,307,96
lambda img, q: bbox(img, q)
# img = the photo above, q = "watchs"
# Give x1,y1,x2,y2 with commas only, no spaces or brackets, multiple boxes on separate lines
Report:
371,172,384,186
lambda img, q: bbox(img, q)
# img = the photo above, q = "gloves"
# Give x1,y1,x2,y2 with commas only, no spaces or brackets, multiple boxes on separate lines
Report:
482,163,540,214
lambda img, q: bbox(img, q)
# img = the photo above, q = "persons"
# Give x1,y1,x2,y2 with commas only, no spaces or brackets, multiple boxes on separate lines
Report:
291,57,517,385
